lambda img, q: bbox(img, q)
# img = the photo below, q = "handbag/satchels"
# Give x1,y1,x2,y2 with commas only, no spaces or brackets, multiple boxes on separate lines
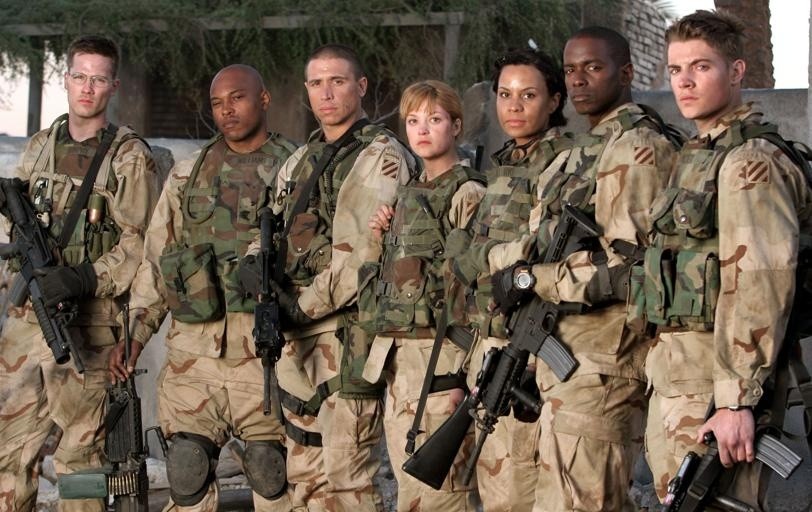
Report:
158,243,224,323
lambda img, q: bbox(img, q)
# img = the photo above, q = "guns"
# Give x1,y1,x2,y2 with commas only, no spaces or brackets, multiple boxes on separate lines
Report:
0,177,84,374
651,376,803,512
252,207,286,415
459,205,604,485
401,315,482,490
56,303,168,511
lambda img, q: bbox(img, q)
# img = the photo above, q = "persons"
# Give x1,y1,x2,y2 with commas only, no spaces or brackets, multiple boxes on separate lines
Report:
643,7,811,512
492,25,685,512
109,65,302,512
0,34,160,512
240,44,573,512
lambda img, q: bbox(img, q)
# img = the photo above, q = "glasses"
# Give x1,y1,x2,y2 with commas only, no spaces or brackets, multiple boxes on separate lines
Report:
68,72,114,87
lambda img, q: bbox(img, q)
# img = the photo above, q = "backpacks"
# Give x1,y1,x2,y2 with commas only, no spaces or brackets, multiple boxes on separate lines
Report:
613,104,690,152
713,122,811,342
41,113,174,196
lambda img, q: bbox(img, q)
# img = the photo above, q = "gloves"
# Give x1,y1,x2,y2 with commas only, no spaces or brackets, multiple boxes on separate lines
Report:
237,255,312,324
42,263,97,308
